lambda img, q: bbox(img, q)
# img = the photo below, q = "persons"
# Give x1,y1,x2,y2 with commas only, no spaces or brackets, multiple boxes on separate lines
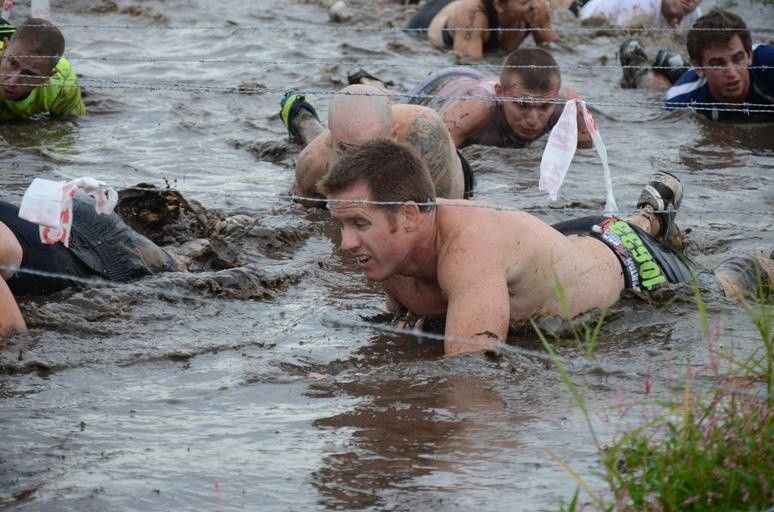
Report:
276,89,476,207
400,1,561,64
618,7,774,126
344,47,599,150
0,181,321,359
0,14,89,119
545,1,703,33
312,138,773,363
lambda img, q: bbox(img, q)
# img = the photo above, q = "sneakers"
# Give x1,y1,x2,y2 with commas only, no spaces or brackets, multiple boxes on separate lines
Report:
651,49,691,85
620,38,648,89
637,171,689,257
347,67,407,92
279,91,320,138
329,1,353,23
